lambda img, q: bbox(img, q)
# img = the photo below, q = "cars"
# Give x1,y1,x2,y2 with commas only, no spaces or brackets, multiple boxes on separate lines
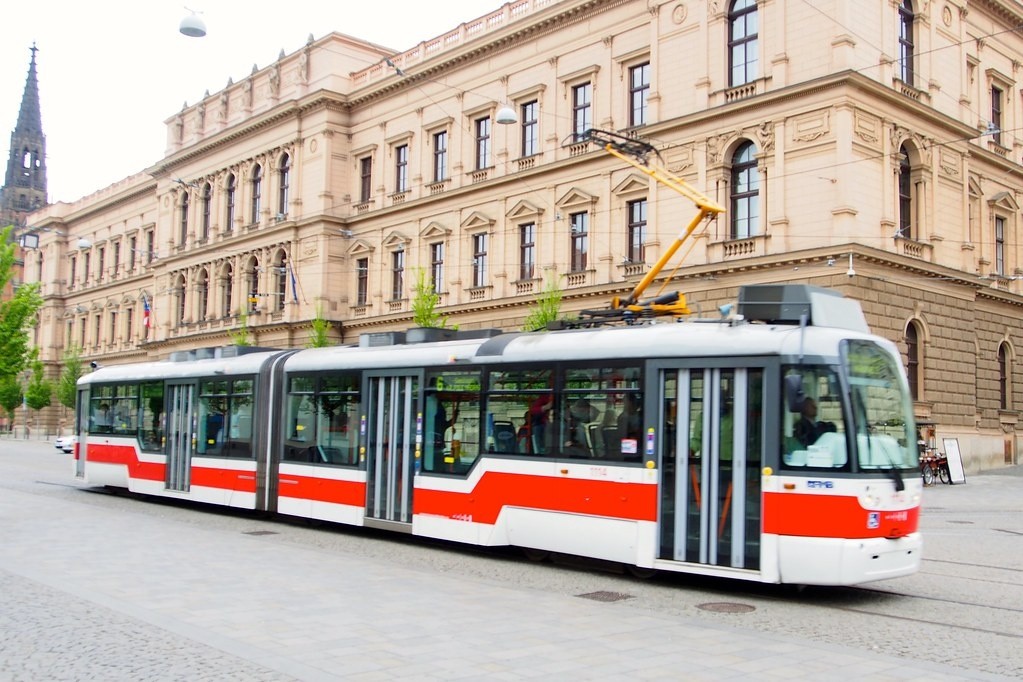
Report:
55,434,77,453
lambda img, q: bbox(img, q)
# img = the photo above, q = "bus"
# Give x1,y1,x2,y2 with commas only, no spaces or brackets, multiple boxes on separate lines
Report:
74,128,924,586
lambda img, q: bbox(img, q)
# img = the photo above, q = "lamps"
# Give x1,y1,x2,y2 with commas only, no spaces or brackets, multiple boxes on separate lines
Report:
496,105,517,124
180,14,207,38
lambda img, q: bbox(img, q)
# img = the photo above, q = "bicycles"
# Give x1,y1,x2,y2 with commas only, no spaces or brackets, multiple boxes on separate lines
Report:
921,446,951,485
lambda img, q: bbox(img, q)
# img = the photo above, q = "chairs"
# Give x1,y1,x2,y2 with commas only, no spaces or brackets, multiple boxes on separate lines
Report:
492,420,623,461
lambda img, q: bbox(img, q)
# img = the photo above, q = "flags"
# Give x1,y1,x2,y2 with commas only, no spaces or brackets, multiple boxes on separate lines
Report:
144,300,149,327
290,264,297,301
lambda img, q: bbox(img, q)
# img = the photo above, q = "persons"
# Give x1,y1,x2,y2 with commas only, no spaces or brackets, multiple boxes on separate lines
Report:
794,398,820,447
519,410,530,434
531,373,570,448
100,404,110,432
416,393,460,474
617,392,640,455
